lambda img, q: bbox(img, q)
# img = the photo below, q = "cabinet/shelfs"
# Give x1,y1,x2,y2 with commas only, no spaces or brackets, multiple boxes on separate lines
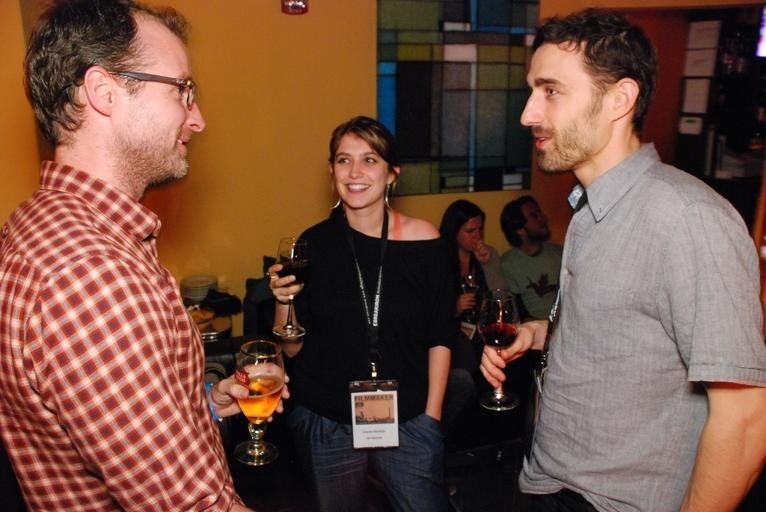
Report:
673,6,766,236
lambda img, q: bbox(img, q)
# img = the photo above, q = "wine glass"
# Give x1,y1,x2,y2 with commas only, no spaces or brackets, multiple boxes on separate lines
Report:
461,274,520,410
272,237,310,339
231,339,286,466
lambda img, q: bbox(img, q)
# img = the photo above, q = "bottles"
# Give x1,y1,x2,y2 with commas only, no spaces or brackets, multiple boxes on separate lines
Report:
745,105,765,150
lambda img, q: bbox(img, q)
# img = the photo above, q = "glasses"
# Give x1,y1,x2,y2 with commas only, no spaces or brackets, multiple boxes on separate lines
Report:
78,71,196,110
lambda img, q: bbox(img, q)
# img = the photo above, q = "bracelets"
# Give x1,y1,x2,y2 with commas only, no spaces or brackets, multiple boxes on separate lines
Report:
205,381,223,425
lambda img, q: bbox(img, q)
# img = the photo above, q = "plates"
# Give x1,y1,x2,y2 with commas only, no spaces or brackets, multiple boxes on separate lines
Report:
180,275,217,302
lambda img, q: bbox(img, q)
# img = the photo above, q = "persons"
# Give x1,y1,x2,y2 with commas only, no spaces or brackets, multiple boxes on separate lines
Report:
0,0,293,511
497,195,564,321
269,115,458,512
439,199,503,343
479,6,766,511
